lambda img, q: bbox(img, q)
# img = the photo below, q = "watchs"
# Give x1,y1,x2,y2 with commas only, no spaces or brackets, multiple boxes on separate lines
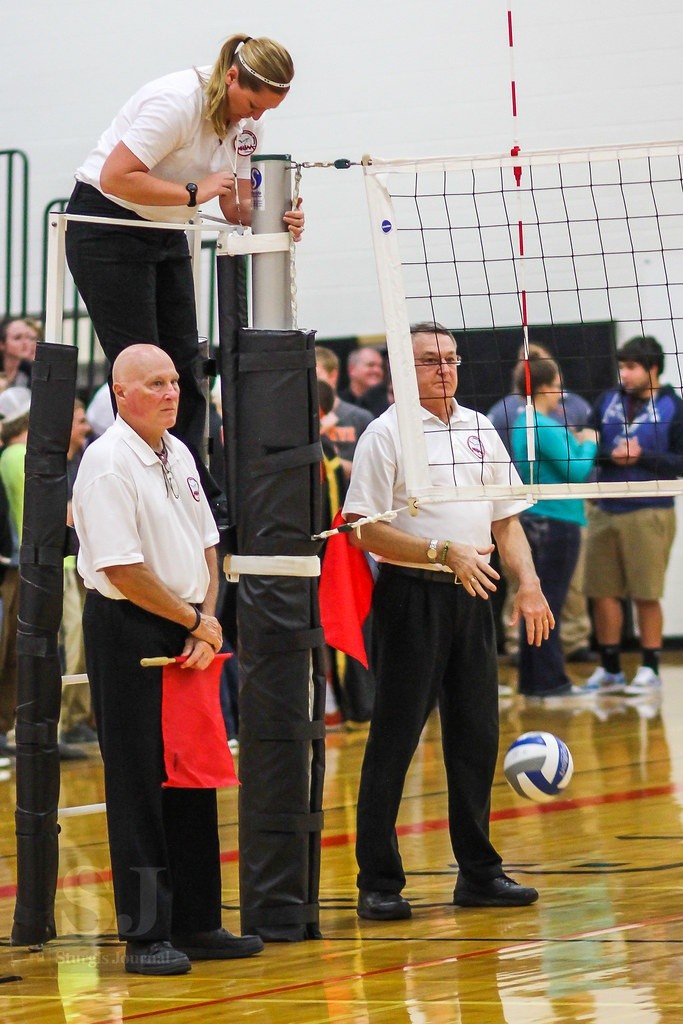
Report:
185,182,198,207
427,538,439,564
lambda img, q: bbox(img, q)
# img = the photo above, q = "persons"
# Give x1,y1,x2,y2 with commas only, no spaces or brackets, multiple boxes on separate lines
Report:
0,320,683,977
64,34,305,520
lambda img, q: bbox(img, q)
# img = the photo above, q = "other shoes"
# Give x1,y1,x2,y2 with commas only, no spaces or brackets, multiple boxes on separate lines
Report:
507,653,518,667
0,746,16,768
60,746,87,761
518,686,593,704
498,682,513,695
227,738,239,747
565,648,596,665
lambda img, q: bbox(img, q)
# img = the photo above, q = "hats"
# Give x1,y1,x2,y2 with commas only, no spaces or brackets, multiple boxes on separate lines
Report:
0,387,31,424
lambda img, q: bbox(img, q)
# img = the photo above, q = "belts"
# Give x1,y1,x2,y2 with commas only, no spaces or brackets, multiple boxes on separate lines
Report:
381,562,462,585
87,588,133,612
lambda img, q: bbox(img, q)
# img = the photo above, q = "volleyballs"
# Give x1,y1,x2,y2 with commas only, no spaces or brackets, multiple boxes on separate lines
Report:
503,730,574,805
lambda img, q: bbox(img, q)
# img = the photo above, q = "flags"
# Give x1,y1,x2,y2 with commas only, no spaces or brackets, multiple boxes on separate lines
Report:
318,509,373,670
161,654,243,789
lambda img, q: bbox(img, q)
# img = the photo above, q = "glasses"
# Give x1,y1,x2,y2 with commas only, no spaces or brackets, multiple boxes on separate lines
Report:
414,354,461,366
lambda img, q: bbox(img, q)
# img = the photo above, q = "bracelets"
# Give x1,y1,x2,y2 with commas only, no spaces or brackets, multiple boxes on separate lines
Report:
441,539,452,567
188,604,201,632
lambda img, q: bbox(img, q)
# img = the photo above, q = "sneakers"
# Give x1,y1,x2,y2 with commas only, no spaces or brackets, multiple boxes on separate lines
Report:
357,891,412,921
581,666,626,693
61,722,99,747
623,668,664,694
454,872,538,908
125,942,190,976
170,928,264,960
326,708,372,730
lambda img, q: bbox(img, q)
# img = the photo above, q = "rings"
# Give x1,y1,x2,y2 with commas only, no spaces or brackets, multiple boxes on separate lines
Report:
469,577,478,582
